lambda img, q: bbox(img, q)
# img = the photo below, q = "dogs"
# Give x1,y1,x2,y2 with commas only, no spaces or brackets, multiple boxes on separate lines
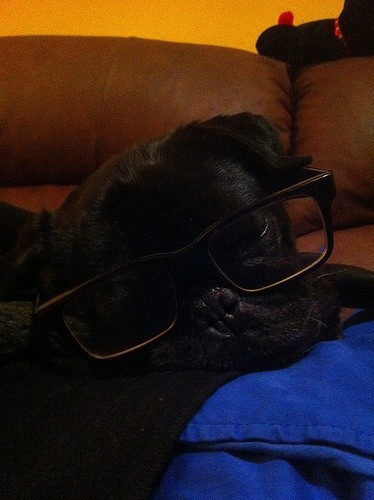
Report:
0,112,349,379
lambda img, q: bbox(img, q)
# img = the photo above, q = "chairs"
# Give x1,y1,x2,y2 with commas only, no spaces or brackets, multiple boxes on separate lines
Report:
0,36,374,335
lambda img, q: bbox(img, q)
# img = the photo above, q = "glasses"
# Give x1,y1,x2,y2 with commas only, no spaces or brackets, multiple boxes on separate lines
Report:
33,165,337,367
